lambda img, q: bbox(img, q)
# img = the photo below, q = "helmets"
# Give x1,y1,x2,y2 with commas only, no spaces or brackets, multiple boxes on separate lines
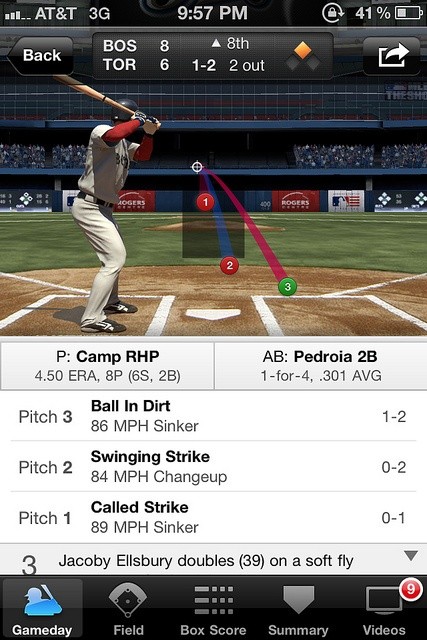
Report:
113,98,137,123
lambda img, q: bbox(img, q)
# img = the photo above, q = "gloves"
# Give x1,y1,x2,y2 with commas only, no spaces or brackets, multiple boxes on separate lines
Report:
141,118,161,136
130,114,147,127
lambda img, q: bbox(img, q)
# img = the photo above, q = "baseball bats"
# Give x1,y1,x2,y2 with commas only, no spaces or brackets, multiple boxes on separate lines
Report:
51,74,156,127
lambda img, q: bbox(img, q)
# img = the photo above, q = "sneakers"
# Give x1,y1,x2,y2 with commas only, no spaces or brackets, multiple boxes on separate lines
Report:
105,300,138,314
81,318,126,333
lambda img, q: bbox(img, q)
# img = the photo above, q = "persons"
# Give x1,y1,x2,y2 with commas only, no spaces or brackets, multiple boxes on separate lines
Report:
0,142,46,169
69,99,161,335
381,143,427,168
51,143,87,170
294,143,375,169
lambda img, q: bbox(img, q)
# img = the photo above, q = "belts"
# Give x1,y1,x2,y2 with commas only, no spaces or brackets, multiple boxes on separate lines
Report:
77,191,115,209
